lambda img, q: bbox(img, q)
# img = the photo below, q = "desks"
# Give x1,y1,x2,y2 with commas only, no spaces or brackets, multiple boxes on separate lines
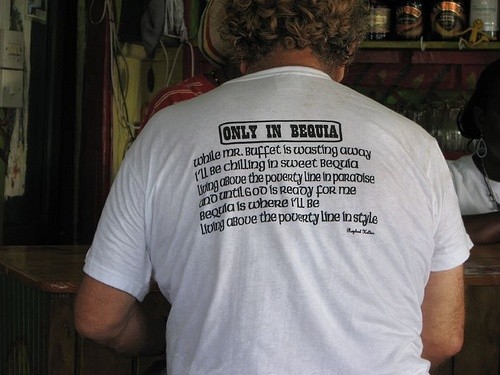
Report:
0,245,500,375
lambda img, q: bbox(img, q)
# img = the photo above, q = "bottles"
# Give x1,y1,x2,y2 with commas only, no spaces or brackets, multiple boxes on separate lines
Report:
430,0,468,42
394,0,424,42
470,0,499,42
364,0,393,40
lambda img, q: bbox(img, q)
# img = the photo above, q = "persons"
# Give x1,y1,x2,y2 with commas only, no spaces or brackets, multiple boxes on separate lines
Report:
448,53,500,247
73,0,474,375
